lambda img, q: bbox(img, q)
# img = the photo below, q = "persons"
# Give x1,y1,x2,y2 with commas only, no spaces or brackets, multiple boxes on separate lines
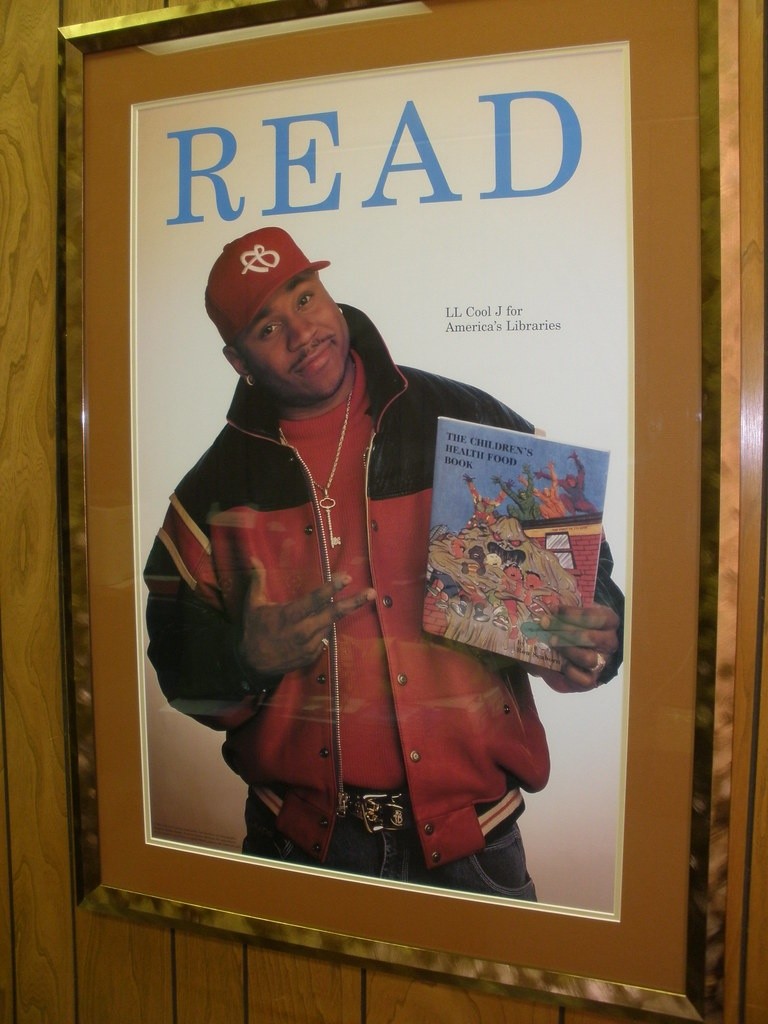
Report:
143,228,625,899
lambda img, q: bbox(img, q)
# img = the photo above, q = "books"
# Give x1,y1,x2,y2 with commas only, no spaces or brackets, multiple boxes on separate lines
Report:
421,416,610,674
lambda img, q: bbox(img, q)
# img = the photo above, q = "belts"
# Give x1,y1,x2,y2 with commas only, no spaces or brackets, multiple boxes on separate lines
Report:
335,789,413,832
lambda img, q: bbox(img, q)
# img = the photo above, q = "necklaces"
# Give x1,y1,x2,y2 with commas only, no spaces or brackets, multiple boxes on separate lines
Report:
278,392,351,548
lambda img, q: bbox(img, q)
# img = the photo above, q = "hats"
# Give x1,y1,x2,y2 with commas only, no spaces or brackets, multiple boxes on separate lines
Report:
203,228,331,346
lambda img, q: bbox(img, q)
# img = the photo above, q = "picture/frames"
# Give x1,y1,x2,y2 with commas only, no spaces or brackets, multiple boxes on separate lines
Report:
54,1,726,1024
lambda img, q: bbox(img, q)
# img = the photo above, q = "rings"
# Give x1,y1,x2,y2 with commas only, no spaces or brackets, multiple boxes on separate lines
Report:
322,639,328,645
589,653,606,674
